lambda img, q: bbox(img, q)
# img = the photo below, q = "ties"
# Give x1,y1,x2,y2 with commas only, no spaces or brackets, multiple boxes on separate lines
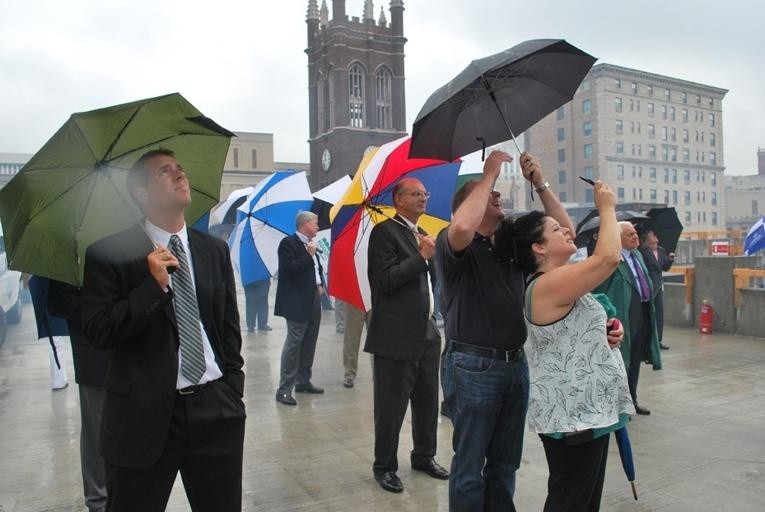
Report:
630,252,649,300
167,235,207,385
412,227,422,246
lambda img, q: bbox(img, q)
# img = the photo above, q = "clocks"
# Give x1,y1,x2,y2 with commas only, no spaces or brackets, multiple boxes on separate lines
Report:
319,147,331,172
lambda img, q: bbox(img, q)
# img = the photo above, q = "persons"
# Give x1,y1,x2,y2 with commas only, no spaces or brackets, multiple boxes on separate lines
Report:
335,297,343,333
434,151,579,512
79,143,248,511
47,277,113,512
594,221,663,422
501,179,636,512
362,177,449,493
341,300,373,387
320,292,335,310
640,230,676,350
274,208,325,406
245,278,273,333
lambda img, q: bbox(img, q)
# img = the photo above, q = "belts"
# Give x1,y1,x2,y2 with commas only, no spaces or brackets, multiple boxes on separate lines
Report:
176,380,211,397
452,340,524,362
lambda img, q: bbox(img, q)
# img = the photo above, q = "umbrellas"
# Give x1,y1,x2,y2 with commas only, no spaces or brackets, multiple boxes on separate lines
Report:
406,35,600,175
0,89,239,292
326,133,465,316
227,168,324,288
310,171,354,273
639,205,683,272
742,217,765,257
576,209,649,236
26,275,81,340
211,185,254,224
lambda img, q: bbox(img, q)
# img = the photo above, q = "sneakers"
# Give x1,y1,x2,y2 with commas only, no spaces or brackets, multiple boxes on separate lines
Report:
258,325,273,330
248,328,254,332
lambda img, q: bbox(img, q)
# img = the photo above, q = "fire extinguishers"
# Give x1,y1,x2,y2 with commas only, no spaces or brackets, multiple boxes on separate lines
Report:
699,299,712,334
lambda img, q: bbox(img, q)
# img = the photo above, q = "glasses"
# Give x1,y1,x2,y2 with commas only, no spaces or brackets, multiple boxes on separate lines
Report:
398,191,431,198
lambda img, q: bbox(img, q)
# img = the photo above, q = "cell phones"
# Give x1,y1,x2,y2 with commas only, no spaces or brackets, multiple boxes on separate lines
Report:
579,176,595,186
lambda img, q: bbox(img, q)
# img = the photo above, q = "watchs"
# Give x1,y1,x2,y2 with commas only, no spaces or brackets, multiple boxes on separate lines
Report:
536,181,549,194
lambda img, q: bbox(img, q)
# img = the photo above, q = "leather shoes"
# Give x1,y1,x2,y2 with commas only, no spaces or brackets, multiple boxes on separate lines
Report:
374,470,403,492
277,394,296,405
659,342,669,349
634,401,650,415
410,451,450,479
344,378,353,388
295,382,324,393
